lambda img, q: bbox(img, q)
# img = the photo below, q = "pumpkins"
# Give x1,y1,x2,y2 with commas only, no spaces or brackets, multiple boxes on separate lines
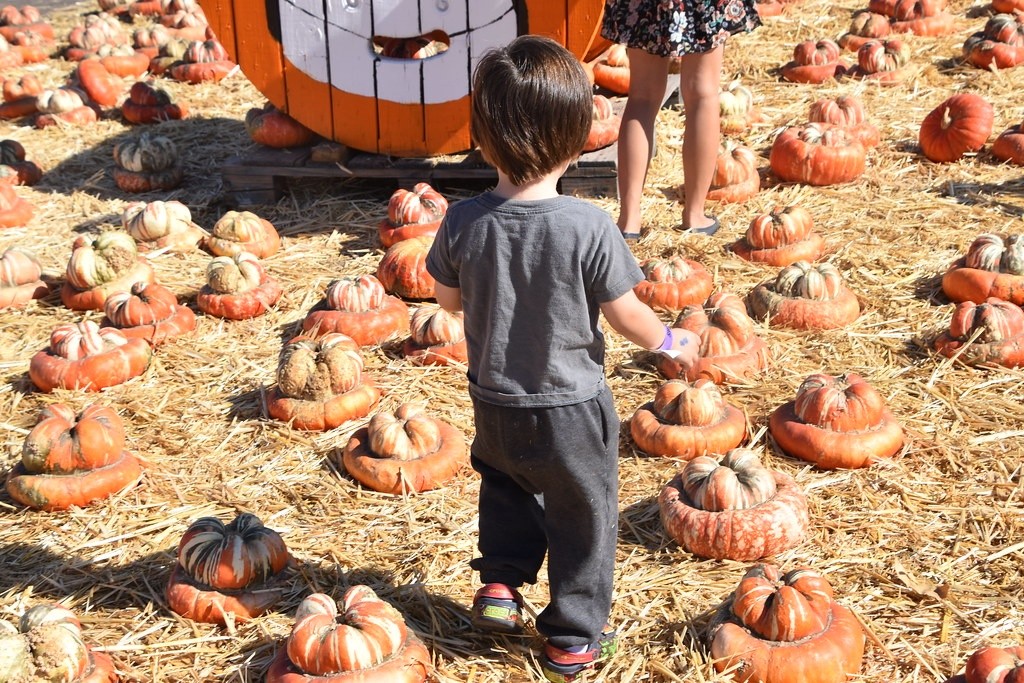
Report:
0,0,474,683
578,0,1024,683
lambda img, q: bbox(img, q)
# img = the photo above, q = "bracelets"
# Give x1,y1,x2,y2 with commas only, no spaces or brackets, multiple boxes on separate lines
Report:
649,327,672,356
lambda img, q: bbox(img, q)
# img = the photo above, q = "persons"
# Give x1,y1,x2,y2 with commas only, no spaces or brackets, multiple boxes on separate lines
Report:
600,0,764,243
426,35,702,677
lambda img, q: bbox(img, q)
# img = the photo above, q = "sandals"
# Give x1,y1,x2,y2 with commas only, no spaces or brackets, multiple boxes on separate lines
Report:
472,583,524,630
541,620,615,683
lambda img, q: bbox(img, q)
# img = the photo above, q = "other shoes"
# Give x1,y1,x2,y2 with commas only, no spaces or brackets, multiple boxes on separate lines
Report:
691,216,719,235
622,233,641,238
